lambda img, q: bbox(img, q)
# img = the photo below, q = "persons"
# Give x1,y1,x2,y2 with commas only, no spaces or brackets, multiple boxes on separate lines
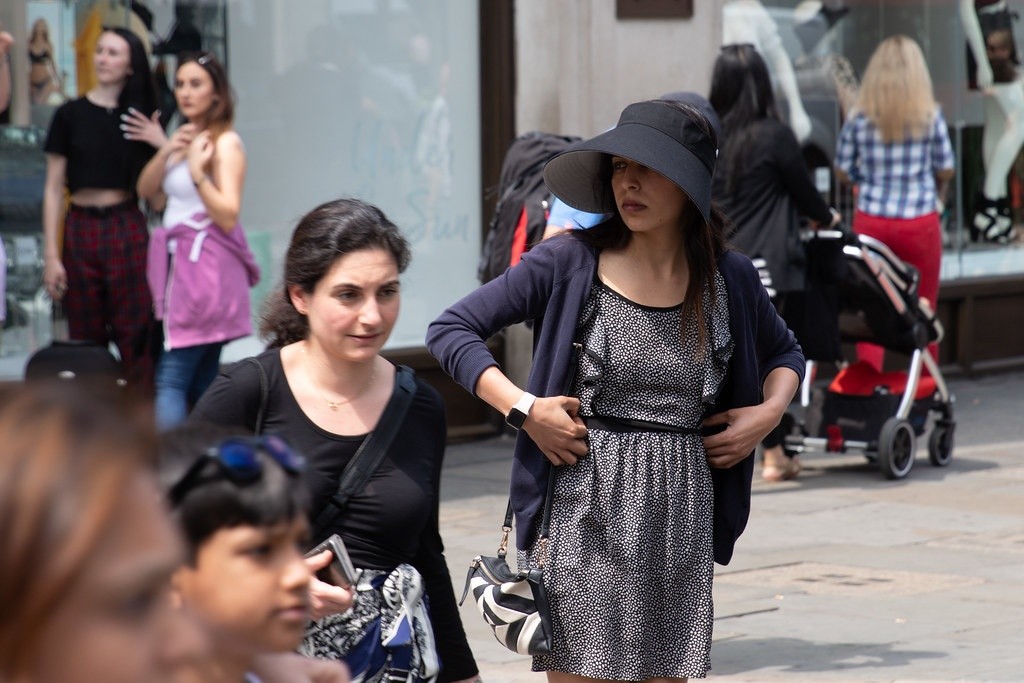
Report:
0,31,16,112
423,90,807,683
169,196,485,683
723,0,861,165
26,18,59,127
0,379,356,683
706,36,956,484
957,0,1024,247
39,23,263,430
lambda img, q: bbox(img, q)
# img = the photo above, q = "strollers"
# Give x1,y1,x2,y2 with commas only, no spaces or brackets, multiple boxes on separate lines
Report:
781,220,958,482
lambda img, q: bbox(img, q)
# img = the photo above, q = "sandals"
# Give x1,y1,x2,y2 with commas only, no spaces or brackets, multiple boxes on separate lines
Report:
762,458,801,484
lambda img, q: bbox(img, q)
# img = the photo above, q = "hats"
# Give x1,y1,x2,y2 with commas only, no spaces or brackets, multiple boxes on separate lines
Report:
543,99,716,225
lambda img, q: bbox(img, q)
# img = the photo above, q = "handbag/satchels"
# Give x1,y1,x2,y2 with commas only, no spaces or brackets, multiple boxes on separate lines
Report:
458,554,554,655
27,287,136,410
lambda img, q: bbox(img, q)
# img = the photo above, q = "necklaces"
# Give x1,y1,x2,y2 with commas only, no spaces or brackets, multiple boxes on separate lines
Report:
105,104,114,115
309,375,376,410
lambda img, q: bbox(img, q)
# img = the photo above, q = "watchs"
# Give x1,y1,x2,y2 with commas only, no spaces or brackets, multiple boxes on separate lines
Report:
504,392,536,431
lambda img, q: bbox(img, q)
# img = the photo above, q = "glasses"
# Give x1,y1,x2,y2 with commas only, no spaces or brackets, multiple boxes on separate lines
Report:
167,435,306,510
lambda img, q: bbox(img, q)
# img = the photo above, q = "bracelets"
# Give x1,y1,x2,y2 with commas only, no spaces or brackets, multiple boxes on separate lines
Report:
195,174,209,189
